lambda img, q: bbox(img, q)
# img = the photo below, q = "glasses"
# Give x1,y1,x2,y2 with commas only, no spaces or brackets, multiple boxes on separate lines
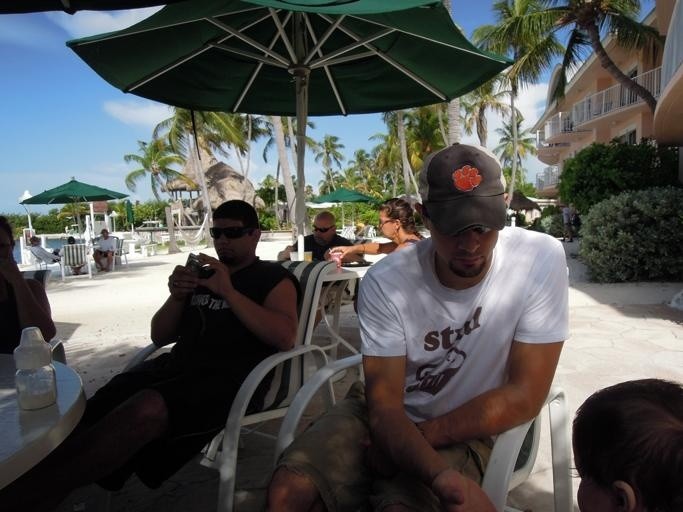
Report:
210,226,251,241
310,224,334,232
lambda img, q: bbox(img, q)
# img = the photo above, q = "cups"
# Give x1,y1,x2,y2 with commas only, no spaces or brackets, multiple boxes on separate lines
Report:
329,251,343,268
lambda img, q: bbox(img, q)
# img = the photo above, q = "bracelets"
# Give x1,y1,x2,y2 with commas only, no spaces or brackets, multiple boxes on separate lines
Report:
429,465,450,487
413,423,424,437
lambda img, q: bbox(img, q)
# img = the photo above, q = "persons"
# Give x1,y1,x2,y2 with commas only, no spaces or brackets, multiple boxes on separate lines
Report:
57,237,83,276
556,202,573,243
565,202,580,240
572,378,682,512
278,212,362,332
91,228,117,273
0,217,56,354
28,236,60,264
264,140,570,511
0,200,303,511
323,198,426,315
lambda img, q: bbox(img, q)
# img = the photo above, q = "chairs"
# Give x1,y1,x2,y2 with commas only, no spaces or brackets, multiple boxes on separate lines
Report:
25,235,126,283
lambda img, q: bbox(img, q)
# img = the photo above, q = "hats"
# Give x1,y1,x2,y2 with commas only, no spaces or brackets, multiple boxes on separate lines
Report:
422,143,508,236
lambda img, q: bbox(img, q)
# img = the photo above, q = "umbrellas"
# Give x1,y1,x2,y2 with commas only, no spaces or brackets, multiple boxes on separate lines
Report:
18,177,130,243
308,187,363,230
338,194,375,227
65,2,516,262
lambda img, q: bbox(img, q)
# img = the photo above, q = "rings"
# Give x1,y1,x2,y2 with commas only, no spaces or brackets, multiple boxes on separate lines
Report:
172,281,179,287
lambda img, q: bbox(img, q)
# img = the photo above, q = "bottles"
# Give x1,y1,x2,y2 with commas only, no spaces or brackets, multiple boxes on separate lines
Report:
14,327,59,411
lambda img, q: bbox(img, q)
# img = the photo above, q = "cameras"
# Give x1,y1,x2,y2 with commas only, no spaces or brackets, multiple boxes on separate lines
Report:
184,253,214,279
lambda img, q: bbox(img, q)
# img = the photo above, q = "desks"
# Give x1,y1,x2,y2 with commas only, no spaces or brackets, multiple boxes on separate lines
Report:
0,350,88,492
